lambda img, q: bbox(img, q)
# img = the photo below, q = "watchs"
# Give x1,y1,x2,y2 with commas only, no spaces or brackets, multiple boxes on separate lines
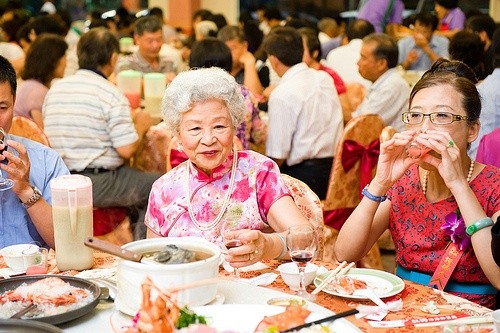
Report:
19,185,42,208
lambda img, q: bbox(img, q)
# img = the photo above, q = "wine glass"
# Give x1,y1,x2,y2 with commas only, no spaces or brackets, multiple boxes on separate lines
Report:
0,127,15,191
286,223,318,302
221,215,249,280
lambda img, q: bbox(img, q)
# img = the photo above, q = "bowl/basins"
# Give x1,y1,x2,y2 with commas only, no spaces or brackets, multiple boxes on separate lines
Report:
490,309,500,333
0,244,39,272
278,262,317,291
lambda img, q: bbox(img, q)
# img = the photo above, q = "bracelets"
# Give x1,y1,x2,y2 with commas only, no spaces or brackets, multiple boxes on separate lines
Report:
273,233,288,260
466,218,493,236
362,184,387,202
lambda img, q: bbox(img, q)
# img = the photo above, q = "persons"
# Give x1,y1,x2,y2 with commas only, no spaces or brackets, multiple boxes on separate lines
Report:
0,0,500,240
333,59,500,310
0,55,71,250
144,67,316,268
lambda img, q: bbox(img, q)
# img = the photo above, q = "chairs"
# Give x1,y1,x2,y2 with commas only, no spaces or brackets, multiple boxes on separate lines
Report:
8,85,396,272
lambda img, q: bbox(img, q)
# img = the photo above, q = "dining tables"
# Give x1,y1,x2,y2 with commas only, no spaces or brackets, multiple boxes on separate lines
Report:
0,251,500,333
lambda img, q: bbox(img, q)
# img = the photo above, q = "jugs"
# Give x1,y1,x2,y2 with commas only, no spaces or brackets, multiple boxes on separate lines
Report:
50,174,94,271
143,72,166,116
117,69,142,110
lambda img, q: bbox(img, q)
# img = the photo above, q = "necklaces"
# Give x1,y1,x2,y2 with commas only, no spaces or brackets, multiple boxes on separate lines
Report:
422,159,475,195
185,147,237,230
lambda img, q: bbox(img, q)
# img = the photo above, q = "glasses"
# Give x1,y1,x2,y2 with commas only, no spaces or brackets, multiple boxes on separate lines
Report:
402,111,467,125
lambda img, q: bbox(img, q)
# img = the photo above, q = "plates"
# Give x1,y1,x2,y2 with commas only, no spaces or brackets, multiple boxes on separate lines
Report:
0,275,109,326
313,268,405,301
190,305,359,333
114,286,225,317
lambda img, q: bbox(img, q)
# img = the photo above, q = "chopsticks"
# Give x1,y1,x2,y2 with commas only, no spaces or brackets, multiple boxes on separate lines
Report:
280,308,359,333
311,261,355,295
8,303,37,318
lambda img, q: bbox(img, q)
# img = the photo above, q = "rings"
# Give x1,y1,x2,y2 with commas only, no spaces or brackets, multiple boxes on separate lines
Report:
250,253,254,261
447,140,454,148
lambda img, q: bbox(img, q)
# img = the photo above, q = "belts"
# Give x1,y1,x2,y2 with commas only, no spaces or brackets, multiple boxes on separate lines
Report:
70,167,110,175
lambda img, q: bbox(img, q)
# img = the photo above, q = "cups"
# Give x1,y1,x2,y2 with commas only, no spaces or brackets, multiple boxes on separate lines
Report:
404,129,431,160
21,247,49,275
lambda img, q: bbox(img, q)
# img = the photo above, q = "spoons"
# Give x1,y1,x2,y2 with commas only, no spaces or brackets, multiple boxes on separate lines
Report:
356,288,388,309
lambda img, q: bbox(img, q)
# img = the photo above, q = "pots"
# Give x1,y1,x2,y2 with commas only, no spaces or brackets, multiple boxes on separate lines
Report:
117,236,221,311
